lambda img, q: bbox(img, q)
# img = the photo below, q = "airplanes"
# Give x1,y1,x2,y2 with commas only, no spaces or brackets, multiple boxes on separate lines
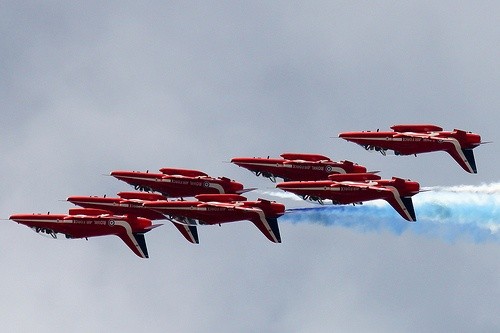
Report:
276,172,421,222
336,123,482,174
231,151,362,182
8,209,163,260
66,193,201,247
109,167,258,200
117,193,285,243
